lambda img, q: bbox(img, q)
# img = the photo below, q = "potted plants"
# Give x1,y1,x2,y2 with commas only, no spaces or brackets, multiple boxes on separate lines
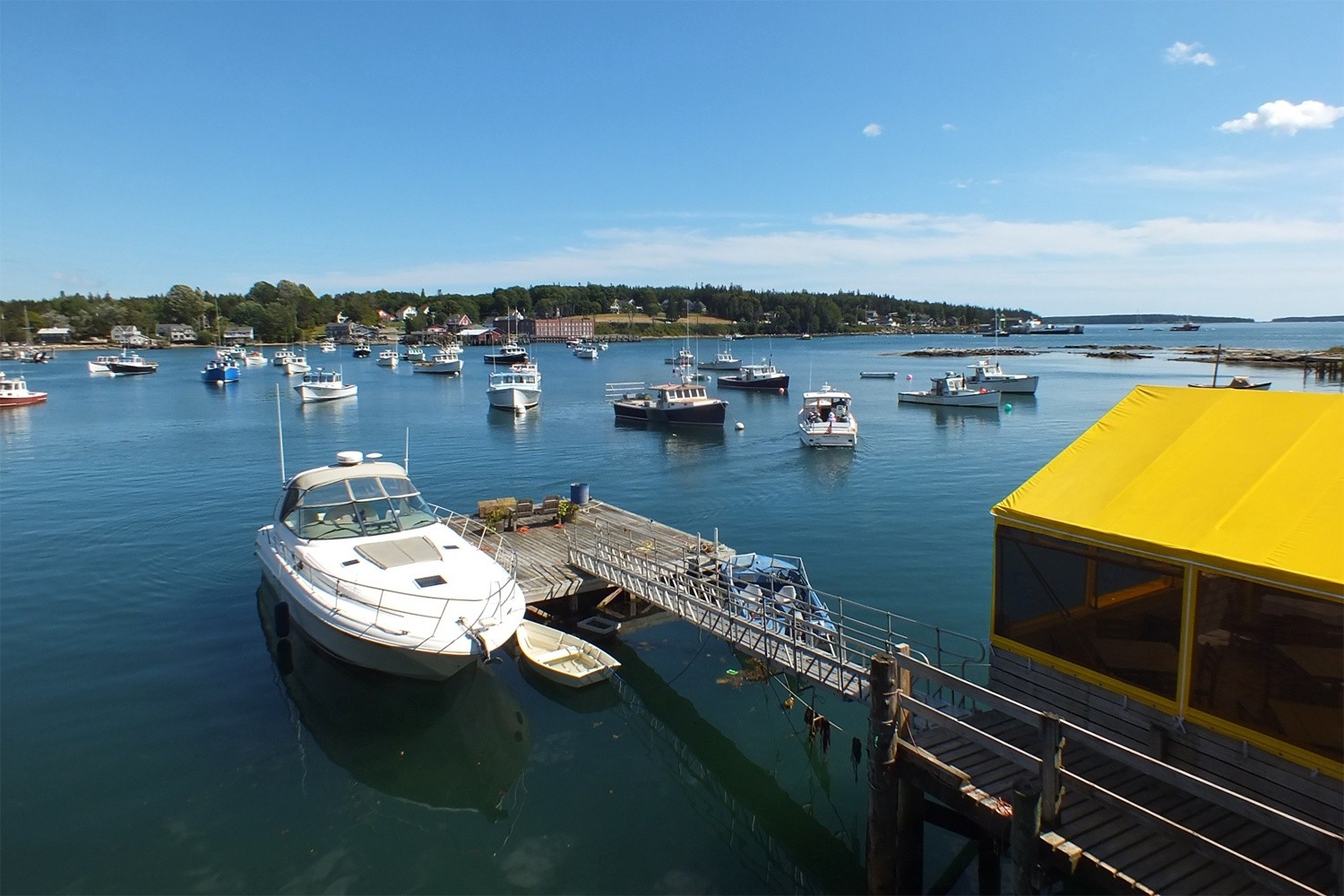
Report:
558,499,579,523
483,506,509,537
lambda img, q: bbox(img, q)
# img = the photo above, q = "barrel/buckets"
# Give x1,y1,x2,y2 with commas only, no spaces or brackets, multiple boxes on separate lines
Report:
570,482,589,506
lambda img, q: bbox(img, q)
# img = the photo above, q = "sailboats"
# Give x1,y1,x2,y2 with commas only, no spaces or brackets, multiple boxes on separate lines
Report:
201,298,242,384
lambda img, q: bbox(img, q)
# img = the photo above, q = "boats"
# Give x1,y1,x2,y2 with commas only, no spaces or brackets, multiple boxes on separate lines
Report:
483,306,528,364
412,348,464,374
353,331,371,358
486,334,542,409
965,312,1040,396
663,299,790,392
87,347,158,376
293,364,358,403
516,618,621,689
0,348,57,364
216,342,312,375
898,370,1002,408
437,337,463,354
252,381,526,684
1170,317,1201,331
406,341,424,361
797,356,859,446
565,338,609,358
518,656,593,710
722,552,837,652
254,572,530,820
982,316,1085,337
0,371,48,407
1187,344,1271,391
375,342,400,366
603,332,730,427
319,338,336,354
859,371,893,378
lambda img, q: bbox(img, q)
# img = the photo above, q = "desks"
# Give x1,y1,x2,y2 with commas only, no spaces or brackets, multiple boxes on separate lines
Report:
1271,644,1344,701
1271,698,1344,748
1094,638,1180,677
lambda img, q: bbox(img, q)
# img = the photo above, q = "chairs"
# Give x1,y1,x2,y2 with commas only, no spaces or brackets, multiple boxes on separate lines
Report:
1097,599,1317,732
740,584,763,602
775,586,797,603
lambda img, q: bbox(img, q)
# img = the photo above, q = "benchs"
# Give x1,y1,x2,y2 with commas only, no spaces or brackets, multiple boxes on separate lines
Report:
508,495,561,532
537,645,580,665
